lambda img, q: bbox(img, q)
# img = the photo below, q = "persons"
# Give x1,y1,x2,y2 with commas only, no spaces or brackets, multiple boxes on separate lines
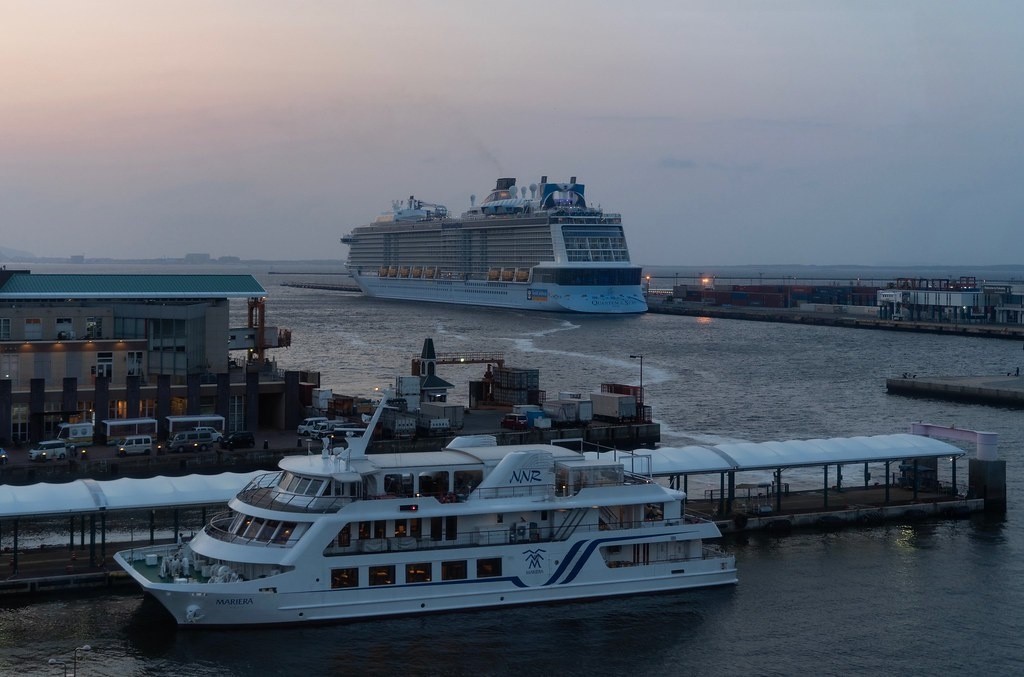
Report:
171,555,180,577
177,533,183,548
181,555,190,576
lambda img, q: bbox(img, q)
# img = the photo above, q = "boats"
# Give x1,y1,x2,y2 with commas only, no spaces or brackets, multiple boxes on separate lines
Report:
113,379,743,629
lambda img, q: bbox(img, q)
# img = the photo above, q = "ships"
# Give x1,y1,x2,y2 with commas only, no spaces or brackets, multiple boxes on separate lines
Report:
338,176,648,315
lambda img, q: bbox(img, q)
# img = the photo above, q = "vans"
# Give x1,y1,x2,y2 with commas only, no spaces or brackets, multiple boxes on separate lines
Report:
164,430,214,454
116,435,152,457
220,430,255,451
331,428,367,441
28,439,66,461
296,418,330,436
192,426,224,443
500,413,529,431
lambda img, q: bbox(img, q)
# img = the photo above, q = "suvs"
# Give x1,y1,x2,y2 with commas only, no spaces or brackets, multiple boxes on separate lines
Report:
319,423,361,443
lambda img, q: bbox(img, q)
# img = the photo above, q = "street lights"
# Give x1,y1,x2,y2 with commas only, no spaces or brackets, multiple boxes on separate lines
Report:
628,355,643,425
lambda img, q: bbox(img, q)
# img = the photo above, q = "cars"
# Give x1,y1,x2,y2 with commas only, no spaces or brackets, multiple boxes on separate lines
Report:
0,447,9,463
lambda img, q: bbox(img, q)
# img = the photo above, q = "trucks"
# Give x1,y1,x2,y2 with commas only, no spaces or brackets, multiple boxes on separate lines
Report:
310,421,344,442
